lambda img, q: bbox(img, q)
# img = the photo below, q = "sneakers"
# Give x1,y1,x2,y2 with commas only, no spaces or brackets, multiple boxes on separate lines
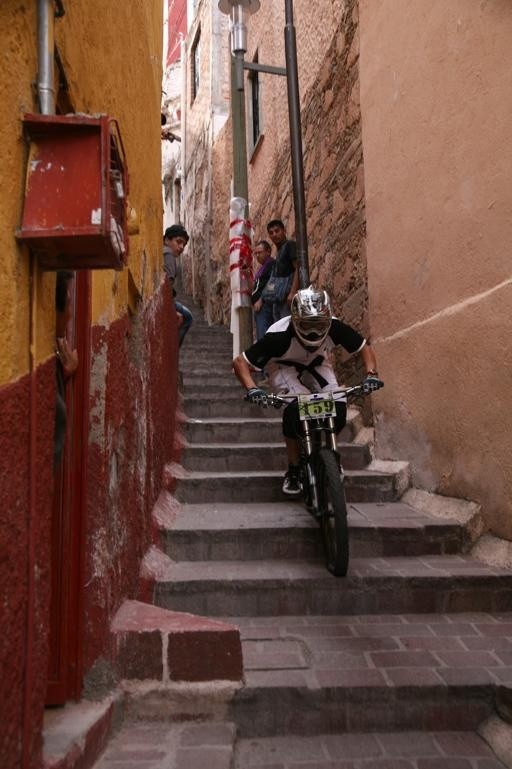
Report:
282,463,303,494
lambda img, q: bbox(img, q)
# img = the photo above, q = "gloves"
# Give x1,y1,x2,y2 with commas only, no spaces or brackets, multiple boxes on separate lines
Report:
247,388,269,408
363,371,379,393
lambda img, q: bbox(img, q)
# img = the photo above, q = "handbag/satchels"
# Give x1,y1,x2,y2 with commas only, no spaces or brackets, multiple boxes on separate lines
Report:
261,263,294,304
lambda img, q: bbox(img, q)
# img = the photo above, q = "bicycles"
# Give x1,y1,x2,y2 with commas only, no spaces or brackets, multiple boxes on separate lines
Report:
255,384,385,578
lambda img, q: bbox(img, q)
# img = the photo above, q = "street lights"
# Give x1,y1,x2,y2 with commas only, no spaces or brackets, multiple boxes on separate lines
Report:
216,0,262,372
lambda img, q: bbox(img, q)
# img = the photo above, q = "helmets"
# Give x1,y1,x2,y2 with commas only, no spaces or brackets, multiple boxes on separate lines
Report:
290,284,332,354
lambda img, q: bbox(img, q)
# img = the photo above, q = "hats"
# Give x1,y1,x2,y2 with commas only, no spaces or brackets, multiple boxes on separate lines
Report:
164,224,186,241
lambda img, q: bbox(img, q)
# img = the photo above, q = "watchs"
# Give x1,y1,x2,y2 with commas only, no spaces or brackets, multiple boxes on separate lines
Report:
367,369,378,375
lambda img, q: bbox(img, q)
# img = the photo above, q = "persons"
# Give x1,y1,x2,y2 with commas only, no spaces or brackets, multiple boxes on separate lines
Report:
234,288,384,495
55,270,79,472
164,224,193,348
250,220,299,340
